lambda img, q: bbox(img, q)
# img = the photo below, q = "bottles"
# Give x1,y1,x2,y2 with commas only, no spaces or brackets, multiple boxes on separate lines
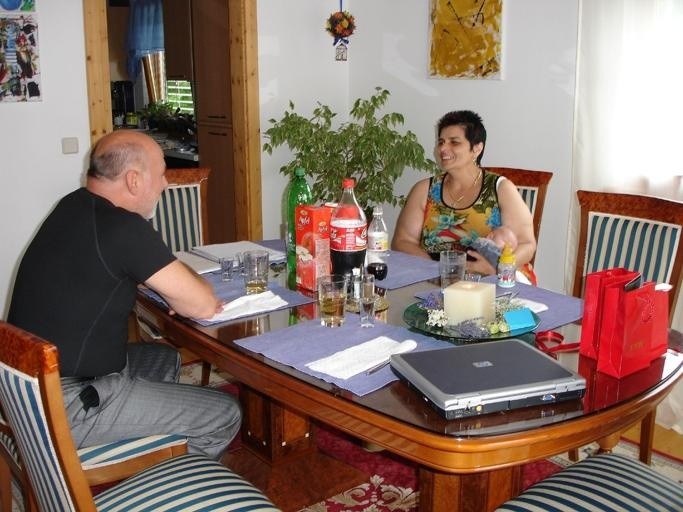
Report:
326,176,368,282
496,244,516,290
365,203,389,282
283,165,313,293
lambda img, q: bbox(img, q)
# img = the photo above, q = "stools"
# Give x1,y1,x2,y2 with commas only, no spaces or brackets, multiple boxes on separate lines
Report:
0,415,200,510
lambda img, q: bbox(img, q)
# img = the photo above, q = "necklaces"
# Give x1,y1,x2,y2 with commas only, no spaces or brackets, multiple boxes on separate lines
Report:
442,171,482,209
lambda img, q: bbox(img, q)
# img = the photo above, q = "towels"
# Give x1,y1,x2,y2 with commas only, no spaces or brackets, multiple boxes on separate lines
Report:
300,333,418,386
198,289,289,325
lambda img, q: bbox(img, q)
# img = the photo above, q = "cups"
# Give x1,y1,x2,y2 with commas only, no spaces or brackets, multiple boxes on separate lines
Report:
216,256,233,284
241,250,269,293
356,272,380,326
235,253,246,277
437,247,468,299
315,274,345,330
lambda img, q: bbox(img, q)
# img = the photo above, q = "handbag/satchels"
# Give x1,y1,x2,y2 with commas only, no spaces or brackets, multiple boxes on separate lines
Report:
578,268,669,379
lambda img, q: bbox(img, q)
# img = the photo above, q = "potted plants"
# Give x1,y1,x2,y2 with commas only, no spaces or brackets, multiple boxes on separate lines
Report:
139,97,174,132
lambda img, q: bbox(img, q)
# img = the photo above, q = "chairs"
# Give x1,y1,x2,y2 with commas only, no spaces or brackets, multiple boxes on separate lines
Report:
480,165,556,270
495,449,682,511
133,164,215,387
0,314,284,512
568,187,682,468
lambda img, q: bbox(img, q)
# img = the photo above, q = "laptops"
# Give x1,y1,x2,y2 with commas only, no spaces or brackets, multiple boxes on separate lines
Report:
392,338,587,421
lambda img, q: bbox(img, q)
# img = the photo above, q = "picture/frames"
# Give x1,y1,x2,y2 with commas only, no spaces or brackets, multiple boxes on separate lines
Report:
425,0,506,84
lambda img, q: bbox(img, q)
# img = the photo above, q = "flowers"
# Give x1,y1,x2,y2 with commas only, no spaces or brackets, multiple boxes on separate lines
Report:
321,9,356,47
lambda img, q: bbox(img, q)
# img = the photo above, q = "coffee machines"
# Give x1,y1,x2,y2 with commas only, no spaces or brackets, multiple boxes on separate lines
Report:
111,81,135,118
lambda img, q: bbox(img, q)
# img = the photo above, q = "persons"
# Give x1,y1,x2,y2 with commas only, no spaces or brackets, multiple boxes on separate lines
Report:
387,108,540,288
5,130,245,461
